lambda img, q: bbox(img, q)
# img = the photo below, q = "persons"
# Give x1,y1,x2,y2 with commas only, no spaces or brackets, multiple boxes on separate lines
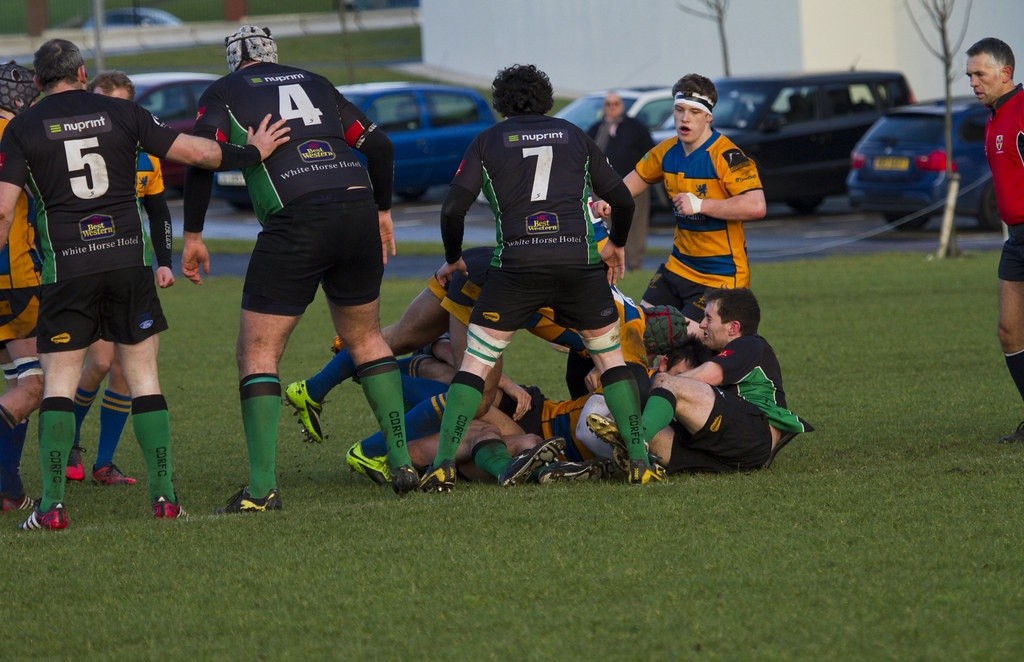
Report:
418,64,669,493
964,36,1024,450
590,70,768,381
284,245,818,486
584,91,655,275
179,23,421,515
0,37,293,531
0,69,174,508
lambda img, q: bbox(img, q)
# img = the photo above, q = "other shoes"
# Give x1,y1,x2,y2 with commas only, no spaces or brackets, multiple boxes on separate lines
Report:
613,446,629,471
586,413,649,455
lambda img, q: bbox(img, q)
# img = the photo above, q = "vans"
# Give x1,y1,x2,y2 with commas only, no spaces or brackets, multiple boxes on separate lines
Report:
650,70,915,222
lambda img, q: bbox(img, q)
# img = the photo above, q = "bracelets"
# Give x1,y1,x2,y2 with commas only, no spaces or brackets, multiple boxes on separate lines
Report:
209,139,260,173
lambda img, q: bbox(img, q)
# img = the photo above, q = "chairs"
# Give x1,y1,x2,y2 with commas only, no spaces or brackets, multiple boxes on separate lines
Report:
783,93,814,124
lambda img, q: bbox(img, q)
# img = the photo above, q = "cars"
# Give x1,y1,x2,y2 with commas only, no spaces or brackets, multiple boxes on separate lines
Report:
211,82,495,210
83,7,181,27
128,71,224,188
847,95,1002,232
554,86,675,132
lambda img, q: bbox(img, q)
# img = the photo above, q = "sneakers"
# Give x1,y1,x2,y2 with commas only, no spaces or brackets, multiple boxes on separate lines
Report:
284,380,323,443
345,441,392,486
420,459,456,492
152,489,189,518
91,464,136,485
533,458,614,484
65,445,86,481
214,484,282,515
628,453,668,485
2,496,35,512
19,498,66,530
498,436,566,488
388,464,419,496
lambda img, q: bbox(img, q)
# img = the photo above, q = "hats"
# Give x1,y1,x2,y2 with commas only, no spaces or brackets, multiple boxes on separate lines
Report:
0,60,41,116
225,25,278,73
